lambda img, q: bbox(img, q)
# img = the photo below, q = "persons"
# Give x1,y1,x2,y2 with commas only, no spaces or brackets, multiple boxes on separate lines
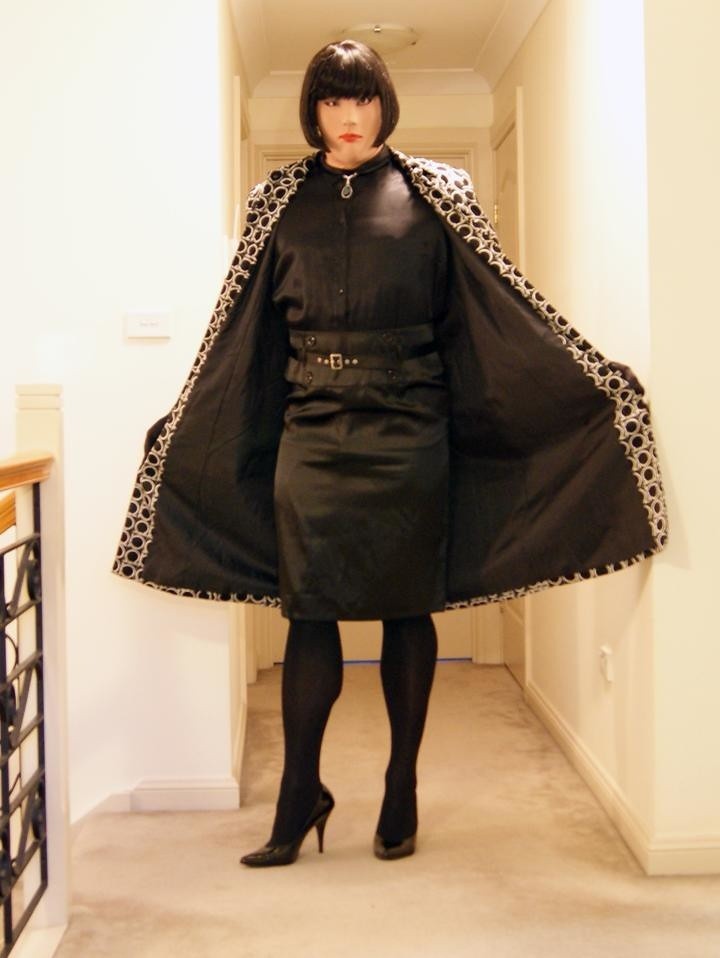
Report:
111,40,668,867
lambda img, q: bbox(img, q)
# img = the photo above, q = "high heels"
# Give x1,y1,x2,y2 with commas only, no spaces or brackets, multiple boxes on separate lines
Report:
373,833,417,859
241,782,336,867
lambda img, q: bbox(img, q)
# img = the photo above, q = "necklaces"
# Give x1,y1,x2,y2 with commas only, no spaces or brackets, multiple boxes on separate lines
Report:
320,148,392,198
